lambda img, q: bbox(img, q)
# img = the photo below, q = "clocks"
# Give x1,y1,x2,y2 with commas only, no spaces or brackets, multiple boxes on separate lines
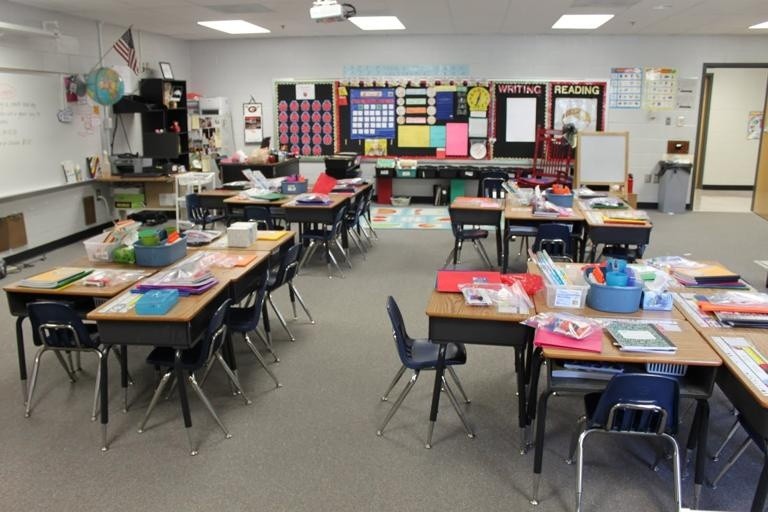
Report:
466,86,491,110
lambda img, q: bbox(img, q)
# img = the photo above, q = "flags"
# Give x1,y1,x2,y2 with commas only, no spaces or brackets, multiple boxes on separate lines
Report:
112,29,138,76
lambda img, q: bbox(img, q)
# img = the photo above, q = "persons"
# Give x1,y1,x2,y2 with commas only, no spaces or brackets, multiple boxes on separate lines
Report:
245,118,261,128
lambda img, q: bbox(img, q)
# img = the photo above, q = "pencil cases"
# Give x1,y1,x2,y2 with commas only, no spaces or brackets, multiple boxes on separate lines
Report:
135,288,180,315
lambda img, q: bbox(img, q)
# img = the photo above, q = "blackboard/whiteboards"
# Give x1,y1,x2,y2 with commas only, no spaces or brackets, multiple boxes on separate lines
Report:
0,67,110,199
273,78,611,163
577,132,629,185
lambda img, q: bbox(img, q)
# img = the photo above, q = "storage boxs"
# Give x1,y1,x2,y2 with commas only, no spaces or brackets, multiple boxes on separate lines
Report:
144,181,175,207
112,195,145,207
373,161,520,181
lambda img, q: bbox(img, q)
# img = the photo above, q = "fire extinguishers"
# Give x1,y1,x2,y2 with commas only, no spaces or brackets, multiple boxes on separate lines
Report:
628,173,633,193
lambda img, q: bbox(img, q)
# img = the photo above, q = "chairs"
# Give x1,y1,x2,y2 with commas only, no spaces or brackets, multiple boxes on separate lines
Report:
377,296,475,442
221,269,283,388
242,243,316,341
23,301,133,422
138,298,253,440
519,123,576,187
561,373,684,510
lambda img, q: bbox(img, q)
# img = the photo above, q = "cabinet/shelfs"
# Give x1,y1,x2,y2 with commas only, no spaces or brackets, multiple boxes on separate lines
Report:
95,176,179,237
139,77,191,169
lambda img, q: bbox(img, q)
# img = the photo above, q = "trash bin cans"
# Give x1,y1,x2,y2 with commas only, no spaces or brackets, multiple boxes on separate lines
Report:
658,160,693,214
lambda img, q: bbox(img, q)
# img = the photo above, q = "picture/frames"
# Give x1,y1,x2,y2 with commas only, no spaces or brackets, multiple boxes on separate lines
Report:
159,61,174,79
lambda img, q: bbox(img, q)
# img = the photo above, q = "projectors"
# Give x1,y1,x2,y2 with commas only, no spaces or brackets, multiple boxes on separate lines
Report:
310,4,348,22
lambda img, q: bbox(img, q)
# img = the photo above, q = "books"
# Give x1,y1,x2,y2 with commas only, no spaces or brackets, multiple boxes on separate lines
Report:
61,160,83,182
527,246,571,288
96,218,140,255
86,155,103,178
532,207,559,218
551,360,624,391
220,169,364,205
672,263,768,329
603,317,679,355
434,265,503,294
128,269,218,296
16,267,121,291
501,179,528,205
580,190,648,224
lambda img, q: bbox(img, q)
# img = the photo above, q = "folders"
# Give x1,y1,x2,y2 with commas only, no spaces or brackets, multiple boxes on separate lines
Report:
248,192,288,202
435,270,502,293
692,293,767,314
533,329,603,353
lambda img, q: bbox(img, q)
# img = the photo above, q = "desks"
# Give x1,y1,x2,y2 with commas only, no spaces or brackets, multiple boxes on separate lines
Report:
185,177,373,280
216,157,300,189
204,229,298,321
528,317,723,506
674,291,766,337
88,273,234,459
447,185,656,274
527,258,670,294
186,229,227,250
423,270,535,450
636,255,760,295
155,249,272,356
4,266,159,382
531,288,687,322
699,330,766,510
64,250,200,271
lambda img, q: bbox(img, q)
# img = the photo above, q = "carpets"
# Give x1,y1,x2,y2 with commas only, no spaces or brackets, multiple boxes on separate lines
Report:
363,205,495,231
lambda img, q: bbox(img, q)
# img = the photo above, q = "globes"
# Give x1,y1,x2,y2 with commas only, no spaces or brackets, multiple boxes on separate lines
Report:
87,66,125,104
